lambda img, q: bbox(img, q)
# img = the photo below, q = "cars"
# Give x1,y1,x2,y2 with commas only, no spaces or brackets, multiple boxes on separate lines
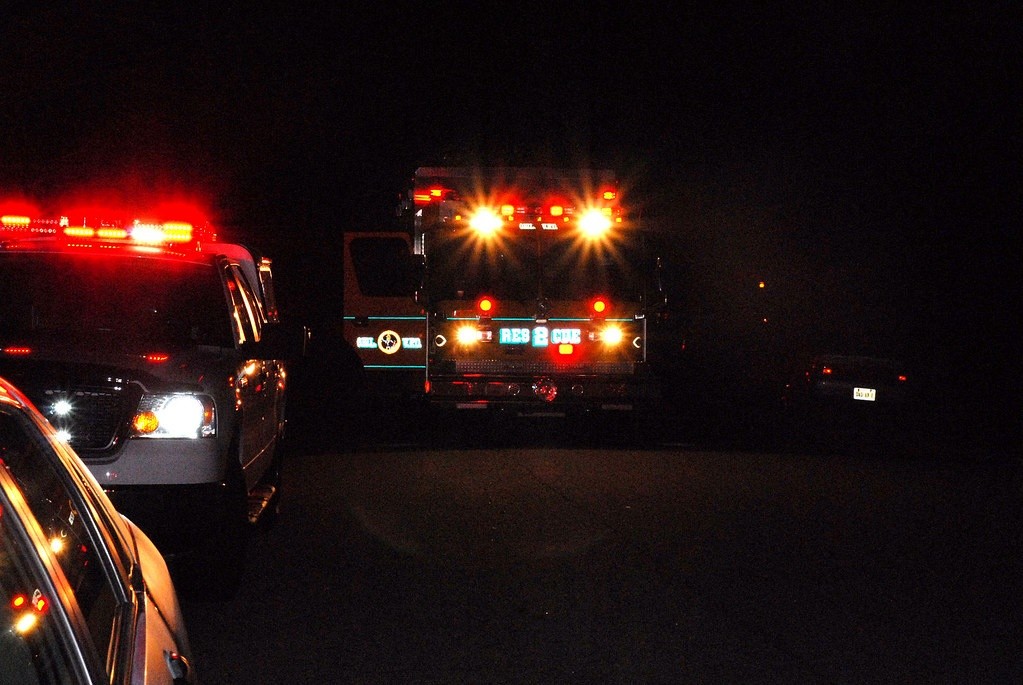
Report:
0,372,198,684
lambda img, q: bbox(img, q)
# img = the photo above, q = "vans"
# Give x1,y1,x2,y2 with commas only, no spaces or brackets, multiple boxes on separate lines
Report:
0,212,308,559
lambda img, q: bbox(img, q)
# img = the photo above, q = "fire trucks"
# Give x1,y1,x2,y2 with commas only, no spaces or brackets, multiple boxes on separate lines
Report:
338,149,665,432
